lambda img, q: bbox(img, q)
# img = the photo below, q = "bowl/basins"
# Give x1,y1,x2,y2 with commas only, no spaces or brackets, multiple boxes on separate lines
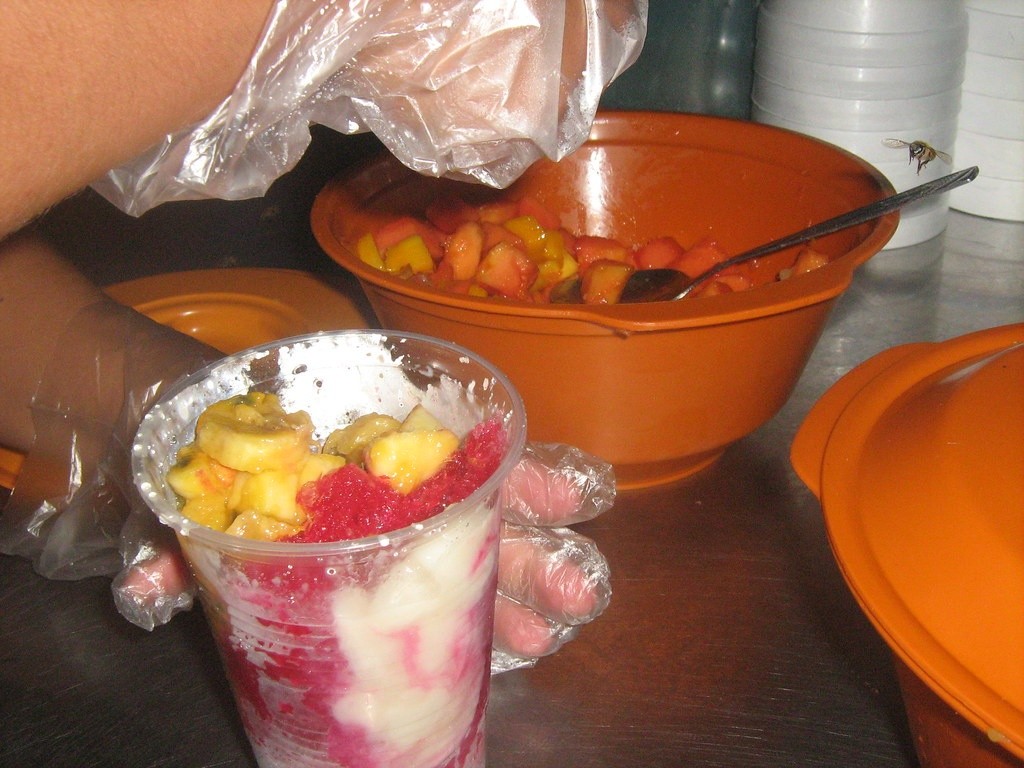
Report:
750,0,1024,252
309,106,902,492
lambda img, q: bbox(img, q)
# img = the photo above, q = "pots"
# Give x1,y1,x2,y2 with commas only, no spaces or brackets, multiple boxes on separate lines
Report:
788,323,1024,768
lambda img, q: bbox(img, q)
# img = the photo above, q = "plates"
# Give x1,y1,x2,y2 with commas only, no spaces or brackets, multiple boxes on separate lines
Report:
0,267,371,518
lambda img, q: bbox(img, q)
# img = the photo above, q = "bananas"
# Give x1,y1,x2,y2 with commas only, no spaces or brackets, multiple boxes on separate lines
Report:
161,390,458,553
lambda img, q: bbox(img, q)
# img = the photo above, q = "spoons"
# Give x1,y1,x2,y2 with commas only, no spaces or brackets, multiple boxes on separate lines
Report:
549,166,981,304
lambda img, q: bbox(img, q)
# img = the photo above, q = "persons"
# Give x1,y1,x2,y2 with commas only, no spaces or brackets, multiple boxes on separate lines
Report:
0,0,649,678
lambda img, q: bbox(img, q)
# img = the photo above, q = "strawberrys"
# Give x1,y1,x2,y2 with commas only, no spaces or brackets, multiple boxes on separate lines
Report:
378,197,828,308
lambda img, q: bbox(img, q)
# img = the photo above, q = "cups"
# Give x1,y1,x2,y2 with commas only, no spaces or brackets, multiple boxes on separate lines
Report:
131,328,528,768
